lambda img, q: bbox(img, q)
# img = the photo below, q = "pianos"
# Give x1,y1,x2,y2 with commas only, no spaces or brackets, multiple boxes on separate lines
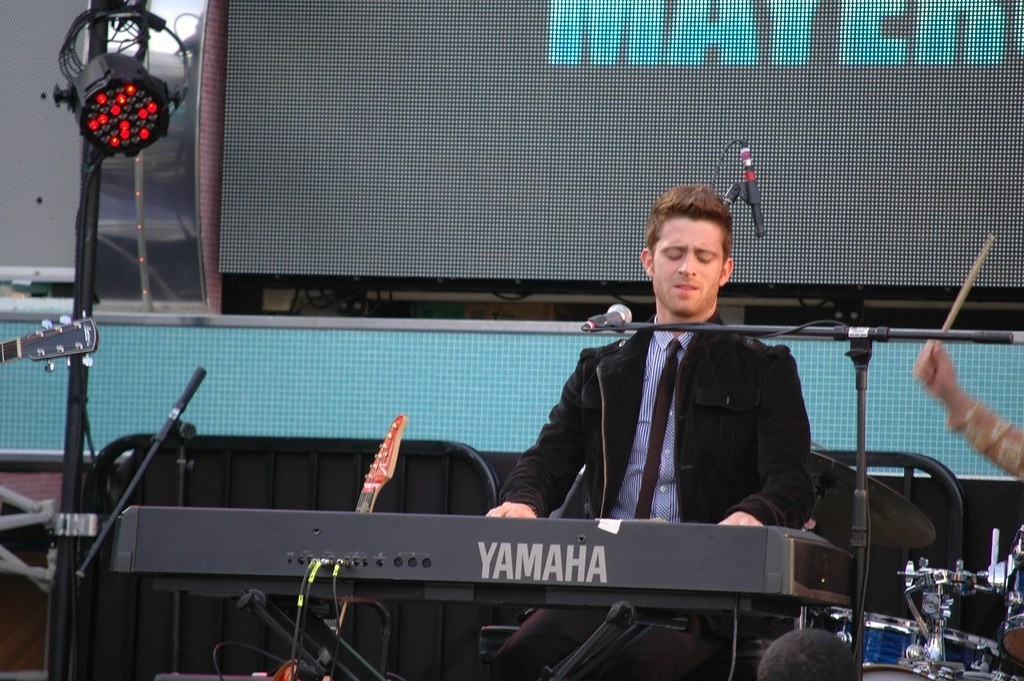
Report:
110,501,856,680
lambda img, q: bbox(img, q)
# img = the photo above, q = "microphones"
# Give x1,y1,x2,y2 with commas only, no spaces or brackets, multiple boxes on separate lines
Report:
581,303,632,333
740,142,765,238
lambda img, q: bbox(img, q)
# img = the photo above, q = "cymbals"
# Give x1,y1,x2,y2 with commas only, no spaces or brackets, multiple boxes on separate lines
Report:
803,447,937,557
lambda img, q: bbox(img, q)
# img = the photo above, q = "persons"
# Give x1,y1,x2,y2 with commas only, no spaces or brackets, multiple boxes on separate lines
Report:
484,185,816,681
913,338,1024,480
757,628,860,681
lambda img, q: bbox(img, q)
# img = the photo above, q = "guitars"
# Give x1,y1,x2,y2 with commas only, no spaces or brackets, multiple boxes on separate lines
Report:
1,309,100,378
267,412,406,680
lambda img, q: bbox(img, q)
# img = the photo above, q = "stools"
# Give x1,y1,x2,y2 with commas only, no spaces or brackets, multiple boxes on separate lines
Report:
473,624,767,681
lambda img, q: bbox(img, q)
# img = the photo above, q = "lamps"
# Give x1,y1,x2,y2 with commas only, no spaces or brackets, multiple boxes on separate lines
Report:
51,54,183,155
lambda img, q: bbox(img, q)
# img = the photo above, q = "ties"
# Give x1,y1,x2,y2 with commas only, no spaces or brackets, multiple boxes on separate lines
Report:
634,339,682,520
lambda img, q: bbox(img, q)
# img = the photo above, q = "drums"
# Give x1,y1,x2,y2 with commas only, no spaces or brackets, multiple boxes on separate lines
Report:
845,527,1024,679
